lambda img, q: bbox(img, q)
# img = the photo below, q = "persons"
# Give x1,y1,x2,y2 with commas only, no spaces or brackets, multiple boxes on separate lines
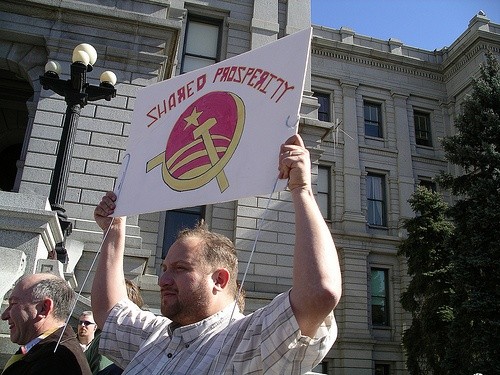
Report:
0,274,95,375
82,279,151,375
90,133,342,375
74,311,98,353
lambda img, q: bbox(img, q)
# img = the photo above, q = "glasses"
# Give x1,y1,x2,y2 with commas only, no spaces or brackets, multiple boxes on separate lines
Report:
77,321,95,325
